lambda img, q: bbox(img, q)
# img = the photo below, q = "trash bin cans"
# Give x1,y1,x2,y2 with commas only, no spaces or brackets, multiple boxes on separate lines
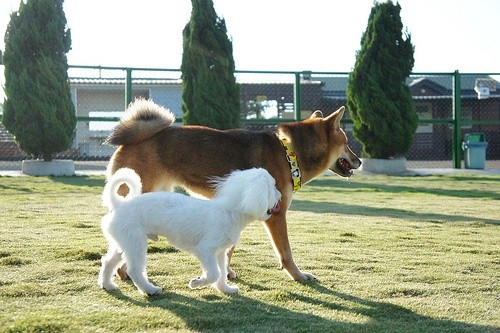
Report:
462,140,489,170
464,131,486,142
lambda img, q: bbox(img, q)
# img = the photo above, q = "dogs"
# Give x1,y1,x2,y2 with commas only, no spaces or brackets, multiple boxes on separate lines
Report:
102,101,361,282
99,167,282,297
